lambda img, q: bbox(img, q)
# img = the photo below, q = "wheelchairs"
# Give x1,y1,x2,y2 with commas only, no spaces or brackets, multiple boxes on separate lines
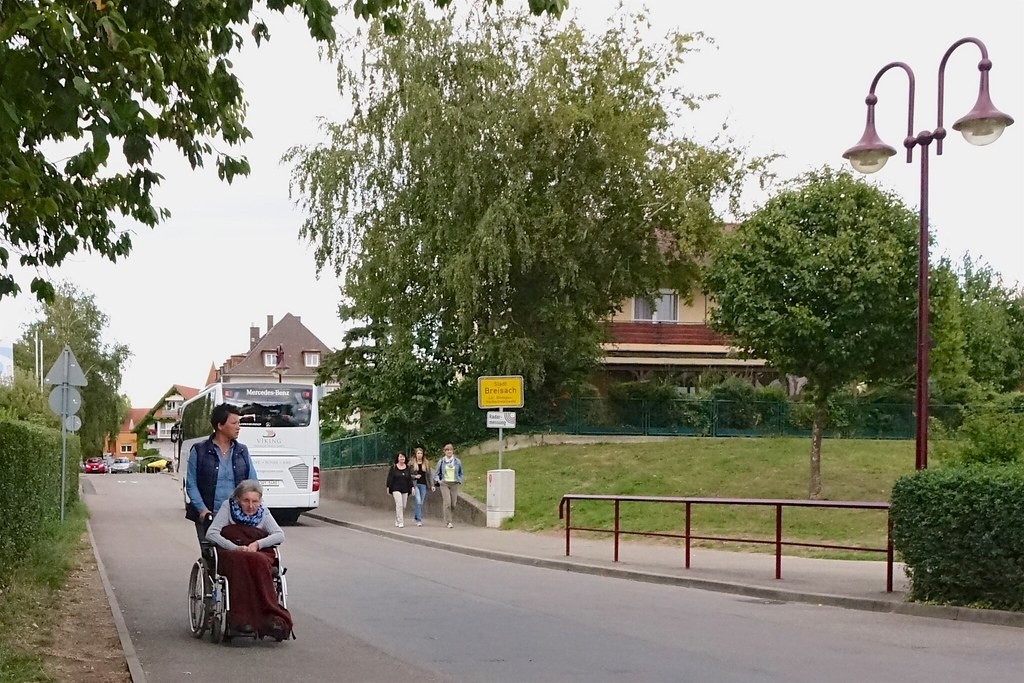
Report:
187,513,298,644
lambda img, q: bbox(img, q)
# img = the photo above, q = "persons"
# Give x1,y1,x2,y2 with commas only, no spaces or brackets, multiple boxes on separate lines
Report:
407,448,436,526
387,451,415,528
433,445,463,528
186,403,258,556
205,479,285,632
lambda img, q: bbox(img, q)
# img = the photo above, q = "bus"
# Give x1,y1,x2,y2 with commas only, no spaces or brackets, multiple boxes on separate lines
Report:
171,383,320,525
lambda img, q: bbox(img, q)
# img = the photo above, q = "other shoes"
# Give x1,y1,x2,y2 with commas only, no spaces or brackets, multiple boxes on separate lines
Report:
445,522,453,528
398,523,403,527
395,522,399,527
415,521,422,526
235,623,254,633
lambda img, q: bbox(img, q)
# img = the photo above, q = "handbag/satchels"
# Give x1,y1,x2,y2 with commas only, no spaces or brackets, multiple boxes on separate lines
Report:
433,461,442,487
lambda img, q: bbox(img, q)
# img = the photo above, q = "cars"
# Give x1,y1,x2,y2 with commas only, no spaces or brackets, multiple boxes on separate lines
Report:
84,456,132,473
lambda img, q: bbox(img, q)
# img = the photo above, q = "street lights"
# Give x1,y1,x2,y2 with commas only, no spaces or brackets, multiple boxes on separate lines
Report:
270,343,291,383
840,37,1015,469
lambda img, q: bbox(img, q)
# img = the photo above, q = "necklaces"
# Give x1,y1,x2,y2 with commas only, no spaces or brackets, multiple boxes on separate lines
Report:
223,451,227,454
